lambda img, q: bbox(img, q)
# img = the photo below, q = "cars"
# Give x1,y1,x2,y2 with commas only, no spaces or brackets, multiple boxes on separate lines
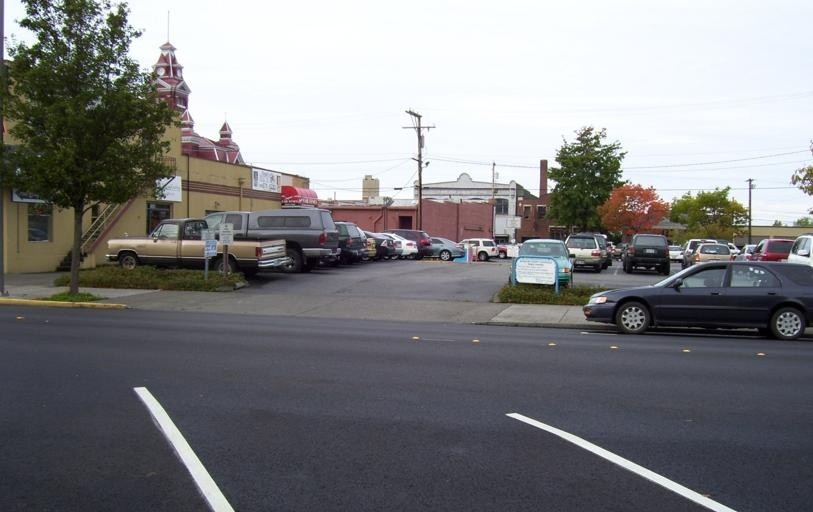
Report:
505,237,576,288
580,257,813,341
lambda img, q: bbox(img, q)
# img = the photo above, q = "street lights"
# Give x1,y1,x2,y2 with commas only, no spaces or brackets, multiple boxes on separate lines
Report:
392,186,419,231
237,175,246,212
492,161,496,201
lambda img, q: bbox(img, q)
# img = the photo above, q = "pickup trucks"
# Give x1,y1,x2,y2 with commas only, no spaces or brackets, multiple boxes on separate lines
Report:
197,207,340,274
102,216,290,275
